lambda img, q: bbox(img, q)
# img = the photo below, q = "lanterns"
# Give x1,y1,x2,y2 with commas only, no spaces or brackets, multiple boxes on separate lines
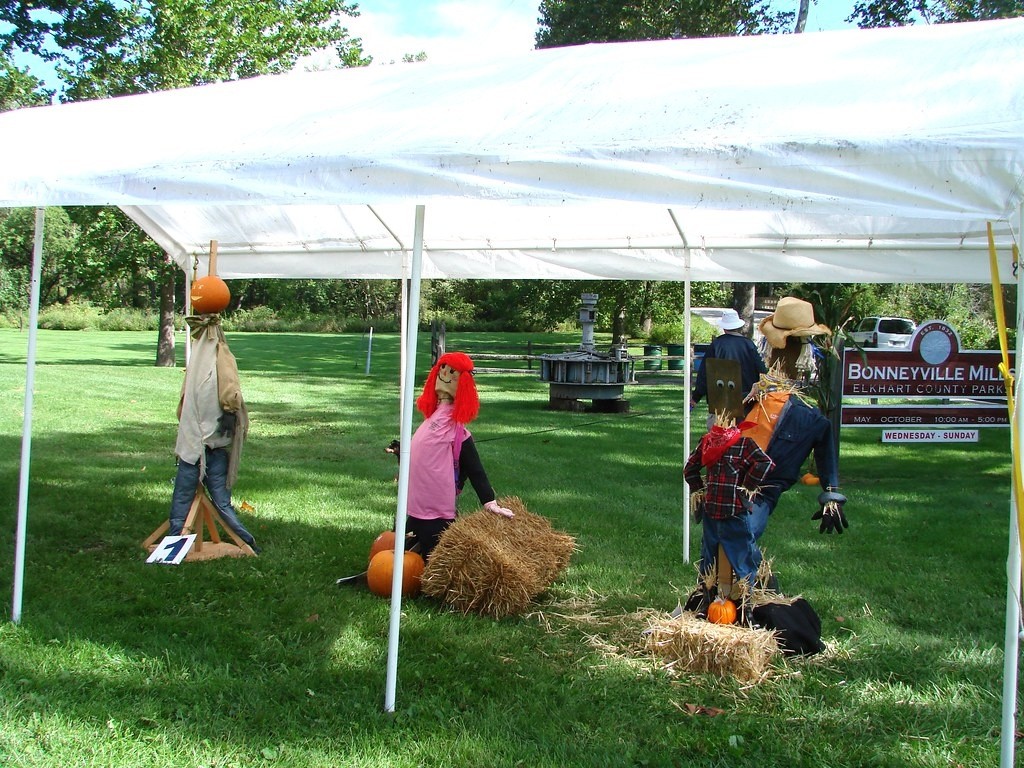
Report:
191,276,230,313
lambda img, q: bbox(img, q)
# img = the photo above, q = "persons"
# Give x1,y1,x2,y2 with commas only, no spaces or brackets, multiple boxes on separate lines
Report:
382,351,516,565
736,295,849,544
689,308,769,434
683,407,782,631
169,313,261,555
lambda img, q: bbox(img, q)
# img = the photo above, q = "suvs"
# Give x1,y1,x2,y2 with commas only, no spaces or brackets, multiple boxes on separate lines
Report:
845,316,917,348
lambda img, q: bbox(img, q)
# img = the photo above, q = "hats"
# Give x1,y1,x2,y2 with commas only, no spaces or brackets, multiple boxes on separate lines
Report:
717,310,746,330
759,297,832,349
